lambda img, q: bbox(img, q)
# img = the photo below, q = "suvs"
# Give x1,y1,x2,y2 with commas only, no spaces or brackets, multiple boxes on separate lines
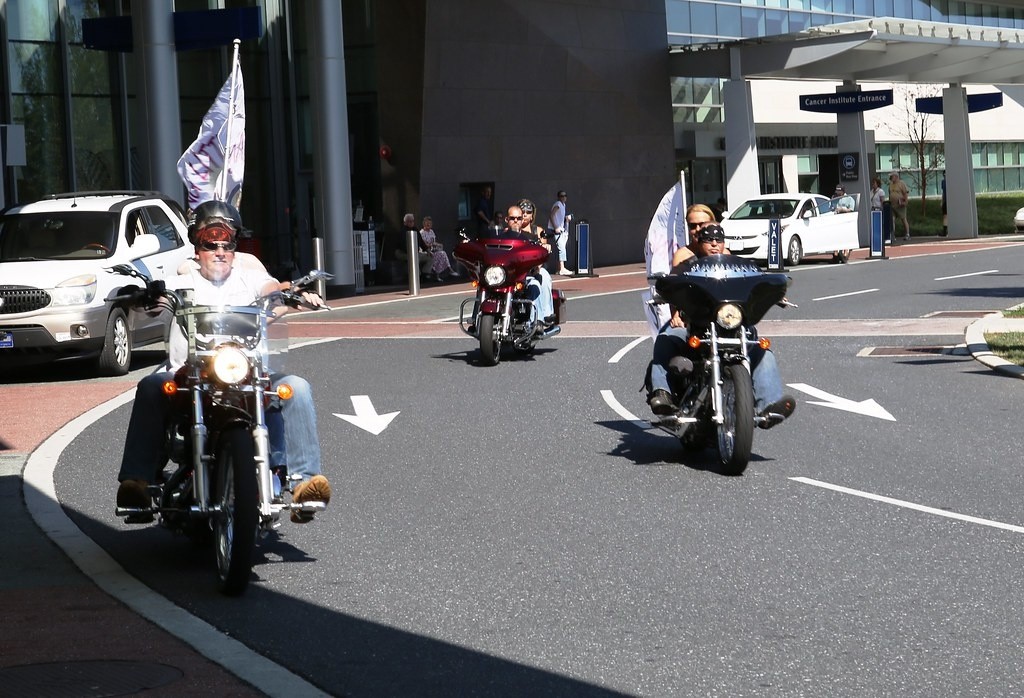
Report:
1,191,194,377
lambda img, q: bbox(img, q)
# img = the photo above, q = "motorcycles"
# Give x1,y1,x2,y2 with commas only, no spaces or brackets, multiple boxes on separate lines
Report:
104,264,337,591
453,225,568,365
643,252,799,476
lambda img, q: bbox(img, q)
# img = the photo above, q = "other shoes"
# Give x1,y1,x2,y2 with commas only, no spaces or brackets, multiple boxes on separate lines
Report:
544,314,557,322
271,465,288,487
829,255,839,264
939,230,947,237
903,234,910,241
443,267,460,278
757,395,796,429
841,256,848,264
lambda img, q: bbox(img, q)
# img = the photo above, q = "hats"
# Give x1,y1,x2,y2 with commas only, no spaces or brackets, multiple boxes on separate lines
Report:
835,184,846,193
698,224,726,245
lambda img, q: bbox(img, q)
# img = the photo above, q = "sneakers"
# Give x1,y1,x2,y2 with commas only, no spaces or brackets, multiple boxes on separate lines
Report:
560,268,576,276
536,321,544,335
116,478,155,524
290,474,331,525
650,389,680,416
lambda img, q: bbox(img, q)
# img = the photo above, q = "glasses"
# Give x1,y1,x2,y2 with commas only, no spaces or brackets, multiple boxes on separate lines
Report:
702,236,724,244
689,223,702,230
201,240,237,252
522,210,533,214
509,216,523,220
559,195,568,198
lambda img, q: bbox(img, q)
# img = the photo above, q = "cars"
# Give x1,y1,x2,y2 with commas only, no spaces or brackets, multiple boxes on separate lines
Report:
1014,207,1024,233
719,193,861,267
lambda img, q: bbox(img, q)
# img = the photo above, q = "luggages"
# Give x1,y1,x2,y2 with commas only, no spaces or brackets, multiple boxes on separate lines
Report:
544,233,561,275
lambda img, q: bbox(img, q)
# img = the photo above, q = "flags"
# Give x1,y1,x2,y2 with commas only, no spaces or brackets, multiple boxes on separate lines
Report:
641,178,689,334
175,60,246,219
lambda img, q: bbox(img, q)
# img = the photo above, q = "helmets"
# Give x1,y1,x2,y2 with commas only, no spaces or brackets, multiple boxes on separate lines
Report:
188,200,243,245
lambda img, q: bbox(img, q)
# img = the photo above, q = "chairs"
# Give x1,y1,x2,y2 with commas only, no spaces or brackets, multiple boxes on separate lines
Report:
21,224,62,256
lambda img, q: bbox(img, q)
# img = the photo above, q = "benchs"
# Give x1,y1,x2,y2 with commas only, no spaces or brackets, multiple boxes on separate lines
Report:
377,258,423,284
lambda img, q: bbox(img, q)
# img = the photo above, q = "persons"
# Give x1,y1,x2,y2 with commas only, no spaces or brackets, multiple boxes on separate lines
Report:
395,213,460,284
466,198,557,337
472,182,505,236
870,171,912,243
547,190,575,276
645,204,796,431
116,199,332,525
938,170,948,237
828,184,855,264
